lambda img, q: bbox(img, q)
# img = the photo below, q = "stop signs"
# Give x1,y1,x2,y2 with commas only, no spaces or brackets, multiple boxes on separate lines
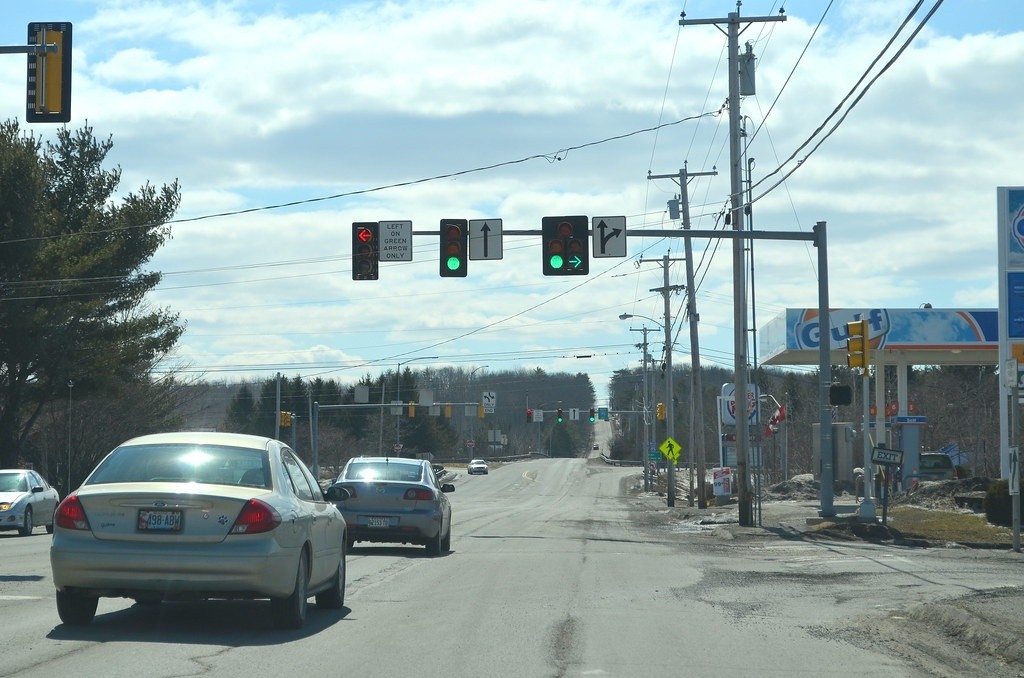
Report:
394,445,401,453
467,440,474,447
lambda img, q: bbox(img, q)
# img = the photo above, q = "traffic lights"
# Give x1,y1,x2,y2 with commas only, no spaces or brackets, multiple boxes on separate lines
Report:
440,219,468,278
846,319,870,368
542,216,589,276
558,409,562,423
590,408,595,422
527,410,531,423
352,222,378,280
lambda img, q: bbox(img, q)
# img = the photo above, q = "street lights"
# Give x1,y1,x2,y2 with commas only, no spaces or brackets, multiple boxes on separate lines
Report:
618,313,674,508
397,357,439,457
469,366,489,462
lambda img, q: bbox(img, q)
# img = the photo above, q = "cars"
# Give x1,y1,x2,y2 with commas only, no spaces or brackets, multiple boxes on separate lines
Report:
50,431,350,629
0,468,61,536
593,444,599,450
326,456,455,554
468,460,488,476
